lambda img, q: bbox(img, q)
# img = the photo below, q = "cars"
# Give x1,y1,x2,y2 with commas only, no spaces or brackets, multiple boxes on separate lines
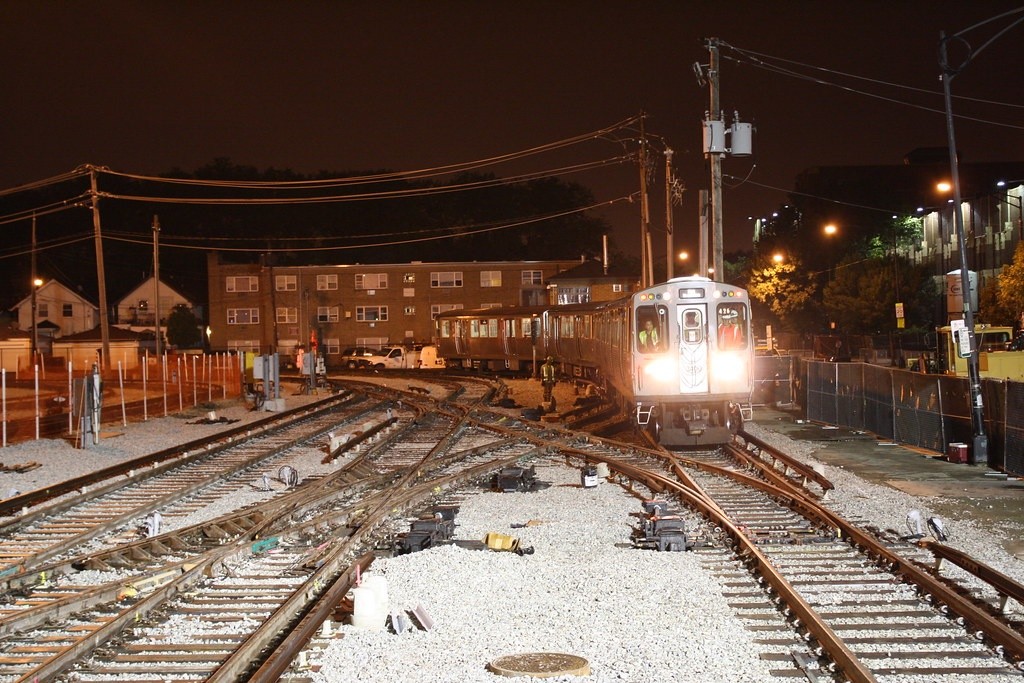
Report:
755,346,784,368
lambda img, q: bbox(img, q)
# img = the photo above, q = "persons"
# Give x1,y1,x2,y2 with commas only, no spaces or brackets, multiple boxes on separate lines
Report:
541,357,555,402
831,341,849,361
718,313,742,349
639,320,659,352
684,312,699,341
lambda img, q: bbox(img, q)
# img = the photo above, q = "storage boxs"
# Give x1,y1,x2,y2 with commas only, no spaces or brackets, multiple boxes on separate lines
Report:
948,442,969,464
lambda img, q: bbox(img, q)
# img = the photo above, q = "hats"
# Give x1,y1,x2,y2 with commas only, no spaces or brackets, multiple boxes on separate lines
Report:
686,312,697,316
721,309,739,319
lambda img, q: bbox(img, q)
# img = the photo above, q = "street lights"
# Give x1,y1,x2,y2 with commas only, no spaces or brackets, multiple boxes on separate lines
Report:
937,179,1023,252
773,252,830,316
825,221,904,367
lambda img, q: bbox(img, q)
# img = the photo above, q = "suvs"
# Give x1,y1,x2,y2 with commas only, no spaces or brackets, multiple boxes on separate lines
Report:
341,346,379,368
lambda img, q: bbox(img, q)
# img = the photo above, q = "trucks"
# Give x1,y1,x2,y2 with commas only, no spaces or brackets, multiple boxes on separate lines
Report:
366,344,443,372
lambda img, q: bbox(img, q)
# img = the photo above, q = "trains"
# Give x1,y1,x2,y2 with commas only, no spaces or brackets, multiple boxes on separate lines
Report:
436,274,754,451
